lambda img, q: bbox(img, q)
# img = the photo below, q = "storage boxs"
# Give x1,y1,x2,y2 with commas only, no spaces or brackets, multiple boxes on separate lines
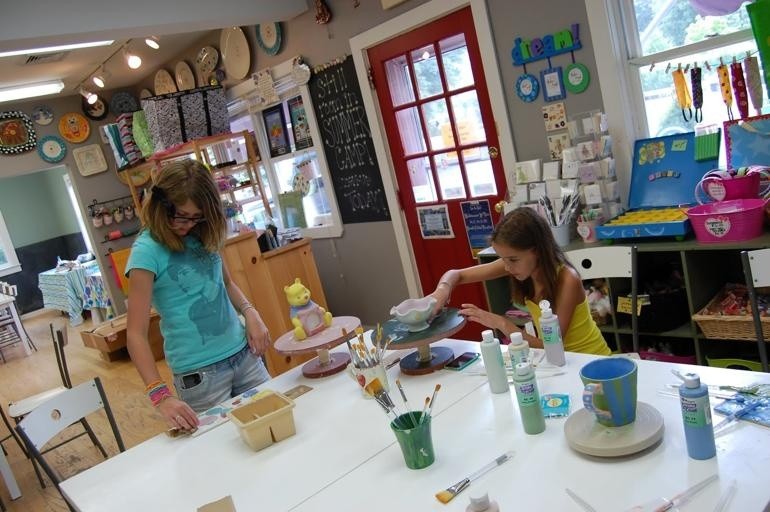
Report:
595,129,721,241
141,84,230,152
79,312,129,353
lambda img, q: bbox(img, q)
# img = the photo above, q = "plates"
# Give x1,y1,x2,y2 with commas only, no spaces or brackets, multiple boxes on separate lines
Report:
58,113,90,142
219,27,251,80
155,69,177,95
37,136,68,164
173,60,195,90
109,92,138,115
114,164,151,186
256,24,282,56
32,103,55,127
81,96,110,122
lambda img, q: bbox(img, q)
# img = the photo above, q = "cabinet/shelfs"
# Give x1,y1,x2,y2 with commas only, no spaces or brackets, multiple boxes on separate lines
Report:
472,232,766,374
111,234,329,381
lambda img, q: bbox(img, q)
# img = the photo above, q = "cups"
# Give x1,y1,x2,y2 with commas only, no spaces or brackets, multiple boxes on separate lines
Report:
391,411,437,469
576,219,597,244
579,356,639,428
550,224,570,248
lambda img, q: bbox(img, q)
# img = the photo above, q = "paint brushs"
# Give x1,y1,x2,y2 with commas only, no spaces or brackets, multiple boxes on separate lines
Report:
380,334,397,360
665,387,746,403
712,401,763,433
437,452,513,503
538,193,580,224
395,379,418,427
578,205,604,223
365,376,407,429
658,474,719,512
422,384,442,424
672,382,761,393
341,328,357,368
351,322,383,369
418,396,431,426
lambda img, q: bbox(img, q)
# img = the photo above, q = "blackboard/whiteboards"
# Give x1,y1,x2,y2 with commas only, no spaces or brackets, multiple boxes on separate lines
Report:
307,54,391,225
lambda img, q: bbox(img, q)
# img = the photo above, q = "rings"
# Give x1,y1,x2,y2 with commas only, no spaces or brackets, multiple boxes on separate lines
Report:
124,157,271,431
174,416,181,421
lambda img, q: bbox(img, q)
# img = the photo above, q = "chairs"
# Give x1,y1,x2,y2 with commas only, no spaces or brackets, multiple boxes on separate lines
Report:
563,246,642,358
0,286,39,364
13,377,127,512
9,332,107,489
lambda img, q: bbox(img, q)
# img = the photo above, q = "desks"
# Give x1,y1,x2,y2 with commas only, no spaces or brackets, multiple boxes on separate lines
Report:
0,295,32,362
83,272,115,326
57,332,770,511
38,259,100,323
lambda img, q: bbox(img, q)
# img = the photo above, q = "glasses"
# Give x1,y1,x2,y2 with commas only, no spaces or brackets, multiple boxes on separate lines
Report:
170,216,201,223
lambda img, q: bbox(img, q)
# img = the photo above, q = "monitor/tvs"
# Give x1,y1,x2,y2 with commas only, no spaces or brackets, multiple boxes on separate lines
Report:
398,204,437,235
416,228,461,250
562,221,597,246
24,246,46,271
201,190,284,248
274,180,355,258
739,213,770,240
379,233,417,262
51,247,97,270
20,145,173,252
668,215,728,243
1,221,21,243
0,244,23,268
362,200,405,232
605,217,660,244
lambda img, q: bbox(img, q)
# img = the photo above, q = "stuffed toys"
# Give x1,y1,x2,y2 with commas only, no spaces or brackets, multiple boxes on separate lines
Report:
283,278,333,340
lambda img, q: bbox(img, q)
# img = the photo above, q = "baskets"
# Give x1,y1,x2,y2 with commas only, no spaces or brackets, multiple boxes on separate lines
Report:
691,288,770,342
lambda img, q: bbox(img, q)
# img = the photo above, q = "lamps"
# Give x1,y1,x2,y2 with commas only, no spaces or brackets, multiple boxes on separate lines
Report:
146,35,161,49
78,87,99,104
121,43,141,70
93,63,112,89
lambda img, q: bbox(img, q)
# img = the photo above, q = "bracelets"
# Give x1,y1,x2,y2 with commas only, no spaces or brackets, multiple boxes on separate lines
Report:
143,379,172,407
438,281,452,305
238,299,254,313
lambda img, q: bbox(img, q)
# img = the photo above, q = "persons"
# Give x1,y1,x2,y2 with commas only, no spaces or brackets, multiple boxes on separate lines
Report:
424,207,612,356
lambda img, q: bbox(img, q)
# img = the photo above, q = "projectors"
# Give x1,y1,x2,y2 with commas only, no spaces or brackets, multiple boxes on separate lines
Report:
359,126,422,147
530,178,567,193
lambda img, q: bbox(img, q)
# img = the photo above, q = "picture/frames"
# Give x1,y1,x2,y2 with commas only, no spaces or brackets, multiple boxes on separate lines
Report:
72,143,108,178
0,109,36,155
